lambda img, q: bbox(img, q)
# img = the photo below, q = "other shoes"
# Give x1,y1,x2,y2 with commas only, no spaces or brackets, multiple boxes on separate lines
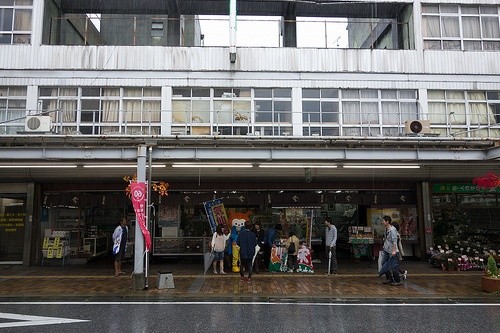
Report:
332,271,337,275
247,277,252,283
240,276,244,281
382,278,393,284
404,270,408,279
213,270,218,274
220,270,227,275
391,280,403,286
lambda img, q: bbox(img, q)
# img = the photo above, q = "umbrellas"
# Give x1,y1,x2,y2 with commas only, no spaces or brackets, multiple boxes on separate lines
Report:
203,247,215,275
379,254,398,279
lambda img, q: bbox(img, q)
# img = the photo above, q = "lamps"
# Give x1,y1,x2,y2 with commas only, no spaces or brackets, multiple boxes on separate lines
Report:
0,162,424,169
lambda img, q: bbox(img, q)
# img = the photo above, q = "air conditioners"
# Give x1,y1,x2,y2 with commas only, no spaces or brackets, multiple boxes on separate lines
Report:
405,120,430,134
25,116,53,131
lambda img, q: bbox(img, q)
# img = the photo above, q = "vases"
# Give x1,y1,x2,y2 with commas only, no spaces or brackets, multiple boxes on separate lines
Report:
457,267,460,272
481,276,500,292
441,263,446,271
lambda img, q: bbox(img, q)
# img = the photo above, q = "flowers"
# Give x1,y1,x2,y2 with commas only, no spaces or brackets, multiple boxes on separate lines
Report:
425,239,500,278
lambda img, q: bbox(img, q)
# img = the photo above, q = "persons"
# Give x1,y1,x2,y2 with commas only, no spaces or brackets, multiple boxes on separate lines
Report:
323,218,338,274
211,224,231,275
256,224,283,274
286,232,300,272
253,222,265,273
112,217,129,277
381,215,408,286
236,221,258,283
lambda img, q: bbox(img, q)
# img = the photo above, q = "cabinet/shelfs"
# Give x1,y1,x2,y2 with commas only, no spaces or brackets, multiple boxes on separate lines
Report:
254,215,279,224
465,207,500,229
56,208,85,232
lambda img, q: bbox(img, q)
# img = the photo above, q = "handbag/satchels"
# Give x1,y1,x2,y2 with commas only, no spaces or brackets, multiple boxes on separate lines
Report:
377,251,387,273
